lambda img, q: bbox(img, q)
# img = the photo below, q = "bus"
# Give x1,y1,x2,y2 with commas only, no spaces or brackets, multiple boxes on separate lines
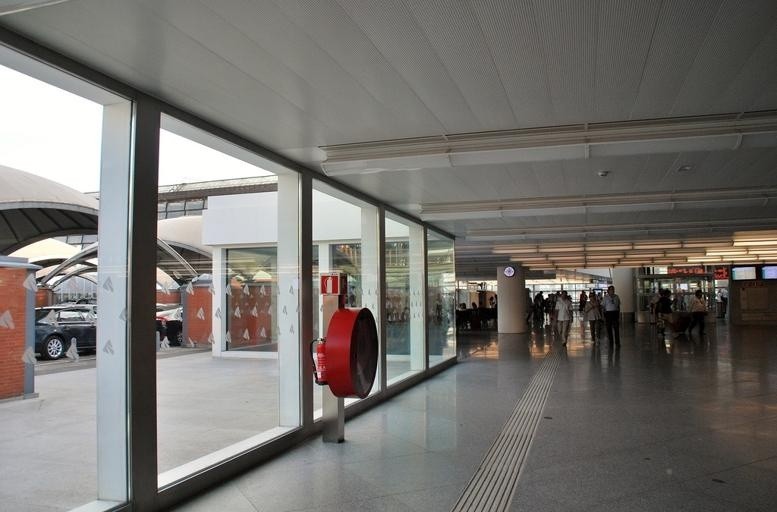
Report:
32,303,185,362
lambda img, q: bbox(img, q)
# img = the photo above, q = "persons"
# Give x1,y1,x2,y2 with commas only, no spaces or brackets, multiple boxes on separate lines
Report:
457,285,728,349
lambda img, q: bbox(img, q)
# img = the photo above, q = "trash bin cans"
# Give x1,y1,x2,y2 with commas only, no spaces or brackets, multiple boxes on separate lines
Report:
715,302,724,318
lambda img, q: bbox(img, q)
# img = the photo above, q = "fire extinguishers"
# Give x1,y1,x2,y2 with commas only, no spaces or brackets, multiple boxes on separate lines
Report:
309,336,328,385
